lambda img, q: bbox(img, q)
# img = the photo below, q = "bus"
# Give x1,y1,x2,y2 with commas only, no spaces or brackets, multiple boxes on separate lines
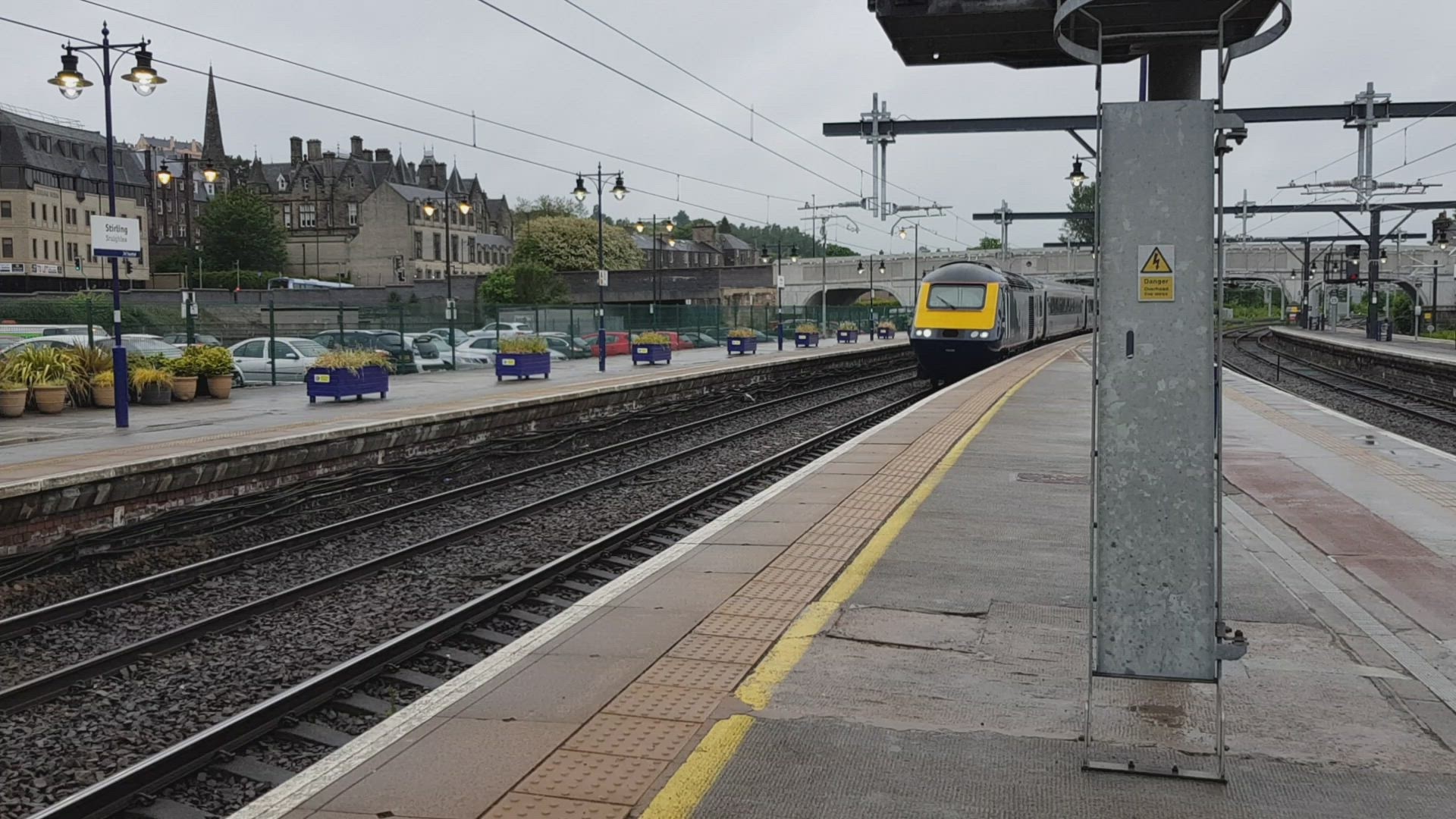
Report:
268,277,354,291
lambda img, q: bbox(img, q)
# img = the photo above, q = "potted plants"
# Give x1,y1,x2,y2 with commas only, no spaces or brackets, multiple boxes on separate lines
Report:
631,331,674,366
837,321,859,343
304,335,400,404
0,335,239,417
726,329,760,356
877,321,897,339
793,322,820,349
492,333,552,381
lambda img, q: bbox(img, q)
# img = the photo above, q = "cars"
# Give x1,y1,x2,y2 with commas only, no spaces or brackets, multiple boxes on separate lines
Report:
225,337,332,382
405,321,567,368
0,324,245,390
683,331,726,348
538,335,594,358
582,332,631,359
707,318,876,346
655,332,694,349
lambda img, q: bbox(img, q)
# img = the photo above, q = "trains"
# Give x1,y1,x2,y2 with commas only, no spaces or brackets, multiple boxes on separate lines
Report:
909,260,1100,382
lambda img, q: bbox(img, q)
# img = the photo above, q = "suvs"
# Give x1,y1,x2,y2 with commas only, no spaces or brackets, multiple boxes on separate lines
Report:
313,330,416,374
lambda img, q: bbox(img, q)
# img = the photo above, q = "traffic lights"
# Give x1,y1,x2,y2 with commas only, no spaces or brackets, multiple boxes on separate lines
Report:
397,272,406,283
396,258,402,270
126,261,133,275
74,256,81,271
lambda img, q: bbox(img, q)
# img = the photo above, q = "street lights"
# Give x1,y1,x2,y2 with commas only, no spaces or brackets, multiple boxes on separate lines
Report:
45,18,169,429
421,184,474,372
635,214,674,332
649,233,676,329
857,254,886,340
571,162,631,368
1289,231,1448,335
157,154,219,348
899,223,919,304
760,240,798,351
